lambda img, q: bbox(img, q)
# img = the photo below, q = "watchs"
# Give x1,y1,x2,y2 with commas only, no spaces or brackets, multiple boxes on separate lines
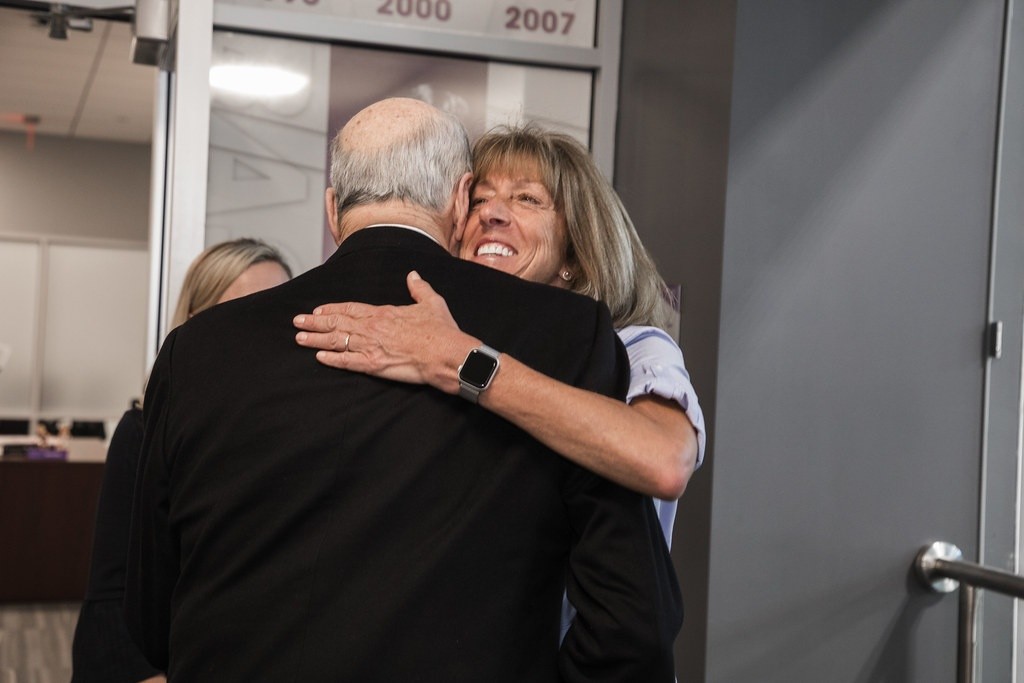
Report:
458,343,501,406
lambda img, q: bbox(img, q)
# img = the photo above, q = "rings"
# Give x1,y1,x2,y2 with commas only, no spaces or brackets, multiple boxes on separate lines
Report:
344,332,352,352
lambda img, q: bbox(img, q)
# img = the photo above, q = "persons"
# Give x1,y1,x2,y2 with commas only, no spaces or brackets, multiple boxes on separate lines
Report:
117,92,686,683
293,122,706,661
64,234,300,683
